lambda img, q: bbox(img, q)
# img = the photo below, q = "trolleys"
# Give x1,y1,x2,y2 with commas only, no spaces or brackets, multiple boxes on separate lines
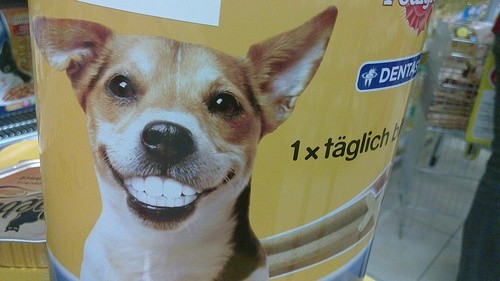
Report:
391,21,498,241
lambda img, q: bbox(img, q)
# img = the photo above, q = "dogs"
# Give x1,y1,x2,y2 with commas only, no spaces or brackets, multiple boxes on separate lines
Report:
31,6,340,281
